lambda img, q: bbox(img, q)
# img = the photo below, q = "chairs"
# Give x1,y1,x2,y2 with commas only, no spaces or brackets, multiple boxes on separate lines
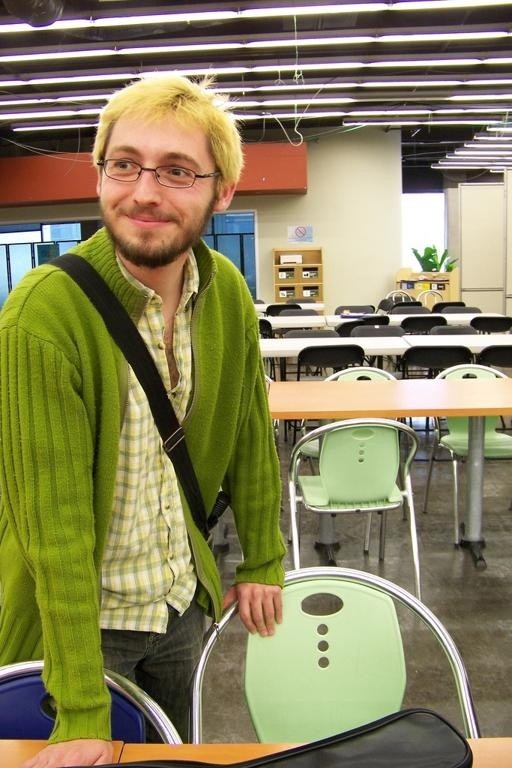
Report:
0,660,179,745
185,560,484,741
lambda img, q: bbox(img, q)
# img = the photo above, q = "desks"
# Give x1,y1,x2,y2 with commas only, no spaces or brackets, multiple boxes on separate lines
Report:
0,734,512,767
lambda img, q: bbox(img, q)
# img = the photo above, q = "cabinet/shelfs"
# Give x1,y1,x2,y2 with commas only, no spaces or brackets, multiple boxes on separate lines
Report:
272,246,324,303
396,268,459,302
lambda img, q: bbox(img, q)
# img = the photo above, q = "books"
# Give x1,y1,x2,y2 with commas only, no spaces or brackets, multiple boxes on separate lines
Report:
401,281,448,290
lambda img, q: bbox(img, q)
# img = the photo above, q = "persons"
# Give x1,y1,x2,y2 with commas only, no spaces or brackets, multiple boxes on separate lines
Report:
0,74,288,767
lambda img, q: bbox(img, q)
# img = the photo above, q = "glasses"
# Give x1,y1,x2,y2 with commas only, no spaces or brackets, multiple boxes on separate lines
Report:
97,159,221,188
